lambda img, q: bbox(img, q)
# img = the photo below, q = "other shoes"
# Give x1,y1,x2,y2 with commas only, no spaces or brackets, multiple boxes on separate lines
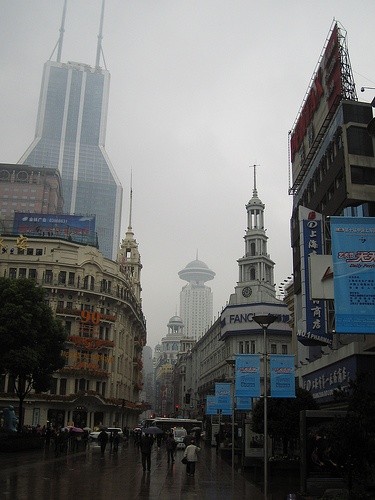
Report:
143,467,146,471
147,468,151,471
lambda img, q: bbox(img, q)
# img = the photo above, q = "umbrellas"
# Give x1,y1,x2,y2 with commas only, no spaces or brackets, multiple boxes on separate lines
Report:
60,426,91,432
191,427,201,430
144,427,159,434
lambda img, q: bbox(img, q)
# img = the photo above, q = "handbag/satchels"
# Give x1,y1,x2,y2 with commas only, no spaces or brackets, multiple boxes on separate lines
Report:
181,454,187,464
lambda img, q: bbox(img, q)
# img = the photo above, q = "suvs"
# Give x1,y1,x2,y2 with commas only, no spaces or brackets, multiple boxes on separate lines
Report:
89,428,123,442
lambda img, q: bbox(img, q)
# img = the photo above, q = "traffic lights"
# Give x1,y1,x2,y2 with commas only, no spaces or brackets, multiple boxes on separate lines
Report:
175,403,179,412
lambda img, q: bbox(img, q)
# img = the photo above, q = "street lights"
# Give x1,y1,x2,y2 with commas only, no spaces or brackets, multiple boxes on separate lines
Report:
251,312,277,499
225,353,236,484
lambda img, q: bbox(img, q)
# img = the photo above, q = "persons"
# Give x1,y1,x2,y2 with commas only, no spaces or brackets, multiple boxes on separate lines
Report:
166,432,177,462
140,433,153,471
109,426,200,450
17,424,90,453
309,431,331,460
98,428,108,454
184,440,200,477
214,429,237,450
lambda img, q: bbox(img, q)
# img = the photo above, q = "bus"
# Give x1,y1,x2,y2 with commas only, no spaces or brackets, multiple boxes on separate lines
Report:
133,417,204,450
